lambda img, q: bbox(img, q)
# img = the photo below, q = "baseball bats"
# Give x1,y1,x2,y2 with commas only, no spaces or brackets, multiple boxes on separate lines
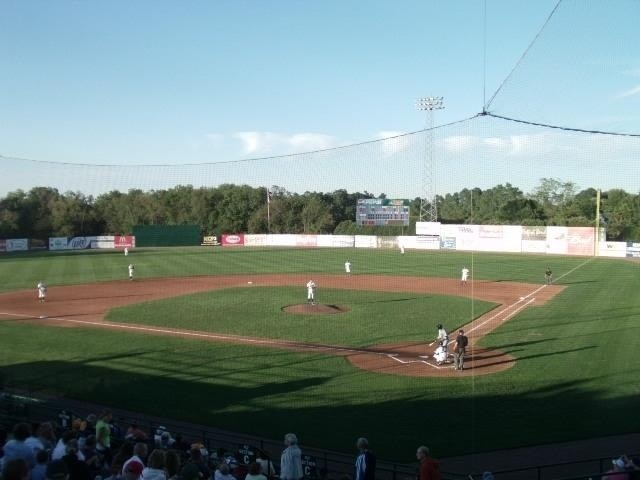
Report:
429,341,436,347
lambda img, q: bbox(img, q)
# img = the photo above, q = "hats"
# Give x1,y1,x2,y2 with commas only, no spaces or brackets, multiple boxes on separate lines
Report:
613,459,624,468
127,462,144,478
87,414,96,422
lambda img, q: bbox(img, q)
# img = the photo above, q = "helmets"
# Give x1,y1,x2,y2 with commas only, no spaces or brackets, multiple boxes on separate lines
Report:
436,324,442,329
439,341,443,345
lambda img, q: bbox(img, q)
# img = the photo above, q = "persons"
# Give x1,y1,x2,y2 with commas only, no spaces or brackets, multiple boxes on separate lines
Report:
127,263,136,281
278,432,305,480
415,445,442,479
601,453,640,480
305,277,318,307
431,322,470,372
460,265,470,287
37,280,48,304
542,267,553,283
342,259,353,276
0,406,278,480
352,436,376,480
